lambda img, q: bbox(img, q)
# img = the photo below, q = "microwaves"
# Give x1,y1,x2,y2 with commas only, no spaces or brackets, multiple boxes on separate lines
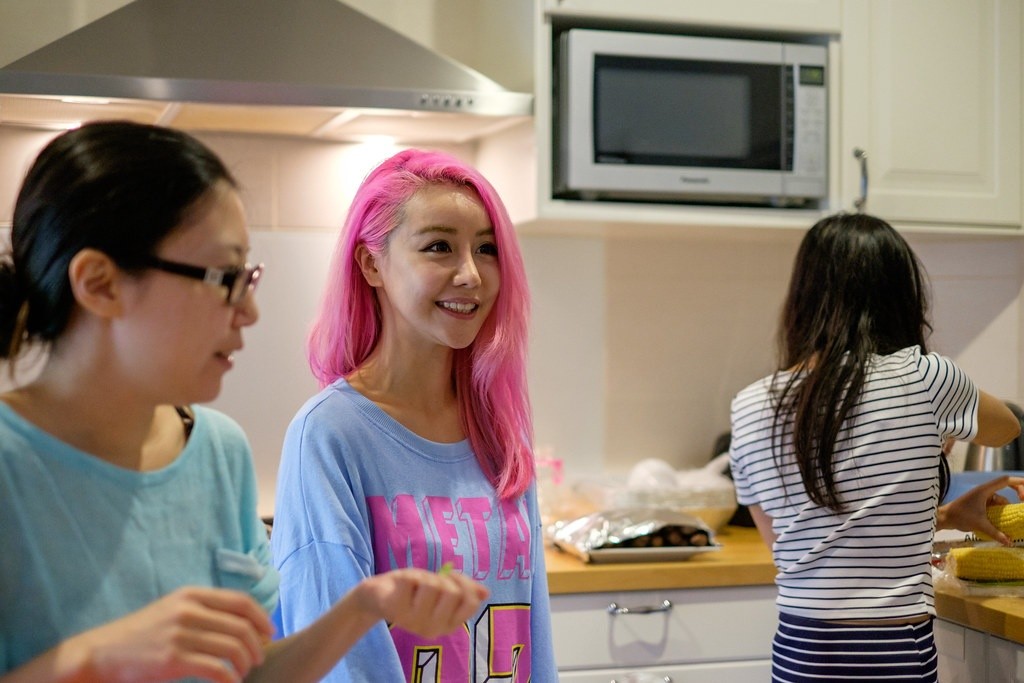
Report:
555,29,827,207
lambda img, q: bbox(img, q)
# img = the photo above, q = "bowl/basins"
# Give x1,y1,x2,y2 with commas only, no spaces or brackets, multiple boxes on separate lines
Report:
684,505,738,534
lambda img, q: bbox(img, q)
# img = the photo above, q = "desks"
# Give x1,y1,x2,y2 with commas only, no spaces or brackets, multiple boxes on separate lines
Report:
543,520,1024,653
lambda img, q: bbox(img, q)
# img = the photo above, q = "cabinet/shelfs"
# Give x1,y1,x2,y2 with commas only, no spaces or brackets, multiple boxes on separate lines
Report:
547,585,1023,682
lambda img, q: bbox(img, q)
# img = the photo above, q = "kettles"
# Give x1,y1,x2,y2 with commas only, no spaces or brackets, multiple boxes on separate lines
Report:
962,400,1024,472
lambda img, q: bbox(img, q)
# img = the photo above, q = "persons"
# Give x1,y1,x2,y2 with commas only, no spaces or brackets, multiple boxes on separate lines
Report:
729,210,1021,682
267,147,557,682
1,118,490,682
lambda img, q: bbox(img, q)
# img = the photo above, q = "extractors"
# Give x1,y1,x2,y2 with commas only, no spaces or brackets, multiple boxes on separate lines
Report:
0,2,537,146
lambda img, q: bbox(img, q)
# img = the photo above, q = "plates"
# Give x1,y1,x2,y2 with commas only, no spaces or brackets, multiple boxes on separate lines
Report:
549,521,720,562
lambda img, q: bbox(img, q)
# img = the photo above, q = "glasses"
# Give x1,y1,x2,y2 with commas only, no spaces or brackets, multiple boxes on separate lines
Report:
142,253,266,308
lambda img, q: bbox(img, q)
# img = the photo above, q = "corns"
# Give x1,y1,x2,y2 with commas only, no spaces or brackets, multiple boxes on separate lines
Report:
974,502,1024,541
946,548,1024,580
